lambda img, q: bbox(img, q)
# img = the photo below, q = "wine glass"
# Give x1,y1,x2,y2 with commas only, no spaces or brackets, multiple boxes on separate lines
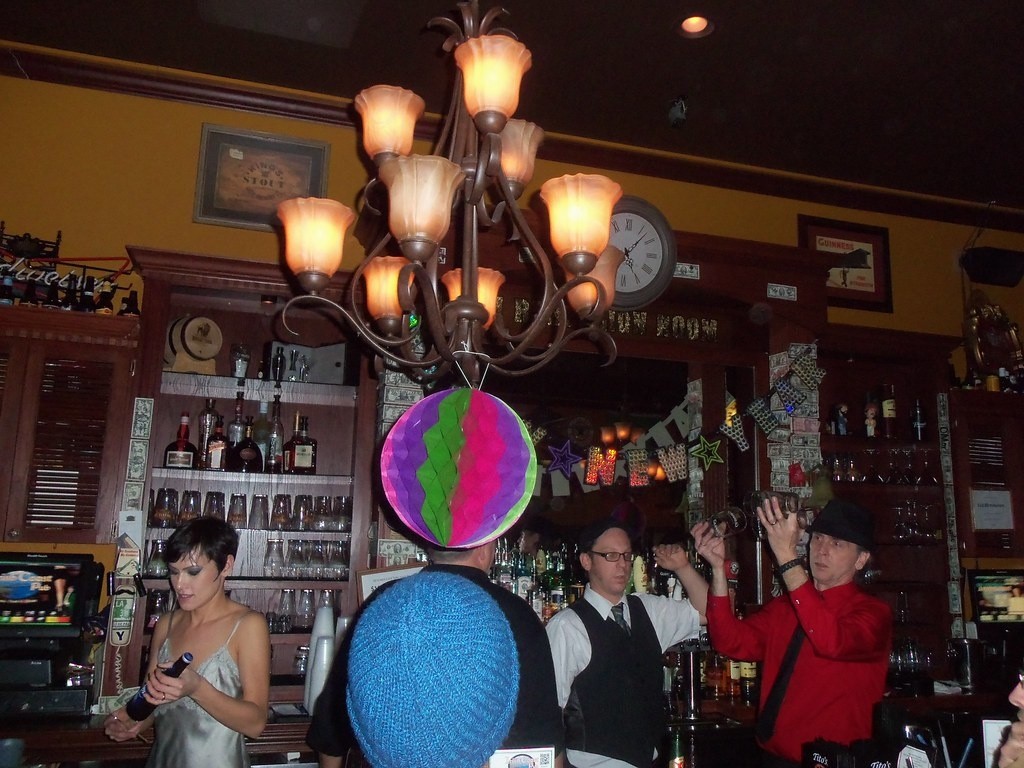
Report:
862,449,940,544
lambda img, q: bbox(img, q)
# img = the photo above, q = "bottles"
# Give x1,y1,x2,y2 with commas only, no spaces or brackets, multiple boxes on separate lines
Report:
829,385,927,440
163,391,317,475
126,652,193,722
669,732,685,768
0,270,140,317
488,536,757,721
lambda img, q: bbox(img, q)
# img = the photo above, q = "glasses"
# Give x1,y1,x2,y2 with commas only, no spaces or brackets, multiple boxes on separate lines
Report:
1016,669,1024,687
587,549,636,562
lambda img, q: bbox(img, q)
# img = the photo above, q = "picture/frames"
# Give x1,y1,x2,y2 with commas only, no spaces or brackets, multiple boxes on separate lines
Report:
192,123,330,232
796,213,894,314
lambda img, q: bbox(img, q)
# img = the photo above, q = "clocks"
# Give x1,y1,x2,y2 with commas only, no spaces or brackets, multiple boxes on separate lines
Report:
597,195,678,309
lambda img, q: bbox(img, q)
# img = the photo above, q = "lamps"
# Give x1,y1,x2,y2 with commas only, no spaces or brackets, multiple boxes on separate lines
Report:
277,1,627,396
673,0,715,39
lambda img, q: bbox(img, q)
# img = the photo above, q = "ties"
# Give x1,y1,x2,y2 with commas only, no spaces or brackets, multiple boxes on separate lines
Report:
754,622,804,743
610,602,631,637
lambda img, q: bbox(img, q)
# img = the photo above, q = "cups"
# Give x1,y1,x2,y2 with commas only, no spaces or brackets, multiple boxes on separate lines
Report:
946,638,983,695
690,489,810,541
230,343,250,378
144,487,351,635
890,591,937,674
292,646,309,682
303,609,354,717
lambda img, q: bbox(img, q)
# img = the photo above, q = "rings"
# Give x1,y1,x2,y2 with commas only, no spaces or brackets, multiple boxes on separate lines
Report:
770,519,778,525
161,692,166,701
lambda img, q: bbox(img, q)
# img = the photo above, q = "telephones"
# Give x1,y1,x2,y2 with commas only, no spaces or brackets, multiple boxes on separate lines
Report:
110,584,136,646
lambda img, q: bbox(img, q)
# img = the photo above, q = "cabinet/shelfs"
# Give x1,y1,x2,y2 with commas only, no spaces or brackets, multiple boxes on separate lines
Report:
0,301,141,546
104,245,384,723
820,324,961,659
950,390,1024,559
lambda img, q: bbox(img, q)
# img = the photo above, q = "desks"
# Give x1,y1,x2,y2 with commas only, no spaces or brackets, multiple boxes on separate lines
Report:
886,664,1014,768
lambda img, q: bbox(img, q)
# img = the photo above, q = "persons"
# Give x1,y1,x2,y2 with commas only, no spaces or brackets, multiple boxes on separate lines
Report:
544,522,711,768
102,514,271,768
515,516,554,556
997,671,1024,768
304,539,567,768
836,403,849,436
690,497,894,768
862,402,877,438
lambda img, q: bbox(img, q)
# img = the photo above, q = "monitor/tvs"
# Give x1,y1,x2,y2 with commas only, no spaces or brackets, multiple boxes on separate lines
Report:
0,551,94,638
968,569,1024,627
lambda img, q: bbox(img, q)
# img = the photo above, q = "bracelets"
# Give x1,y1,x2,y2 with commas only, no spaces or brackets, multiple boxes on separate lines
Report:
777,558,802,576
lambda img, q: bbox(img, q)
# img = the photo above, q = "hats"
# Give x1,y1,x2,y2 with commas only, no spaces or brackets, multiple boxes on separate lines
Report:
806,498,875,551
345,570,519,768
